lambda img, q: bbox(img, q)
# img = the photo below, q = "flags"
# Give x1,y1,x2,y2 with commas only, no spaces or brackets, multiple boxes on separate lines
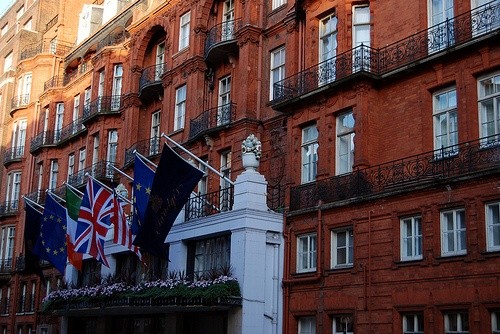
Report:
130,153,156,267
64,184,83,272
110,178,148,267
73,177,125,268
131,141,204,262
23,201,47,288
31,192,68,275
